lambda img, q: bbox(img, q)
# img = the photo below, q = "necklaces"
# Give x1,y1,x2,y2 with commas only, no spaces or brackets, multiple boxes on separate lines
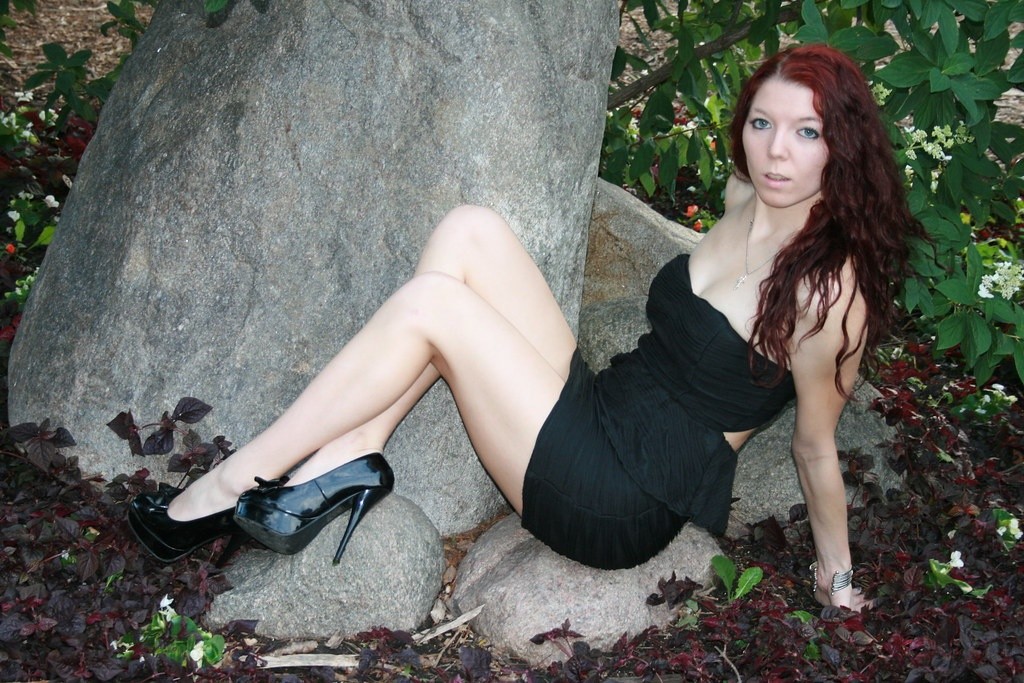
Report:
733,215,785,290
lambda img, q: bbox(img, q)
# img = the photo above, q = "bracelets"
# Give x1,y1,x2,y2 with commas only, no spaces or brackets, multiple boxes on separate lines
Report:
809,561,853,595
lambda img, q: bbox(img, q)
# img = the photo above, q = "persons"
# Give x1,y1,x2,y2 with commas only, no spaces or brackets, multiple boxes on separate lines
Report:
126,45,950,617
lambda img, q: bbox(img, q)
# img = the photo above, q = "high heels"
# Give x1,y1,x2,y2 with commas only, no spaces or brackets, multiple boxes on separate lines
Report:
126,493,242,567
234,453,395,567
